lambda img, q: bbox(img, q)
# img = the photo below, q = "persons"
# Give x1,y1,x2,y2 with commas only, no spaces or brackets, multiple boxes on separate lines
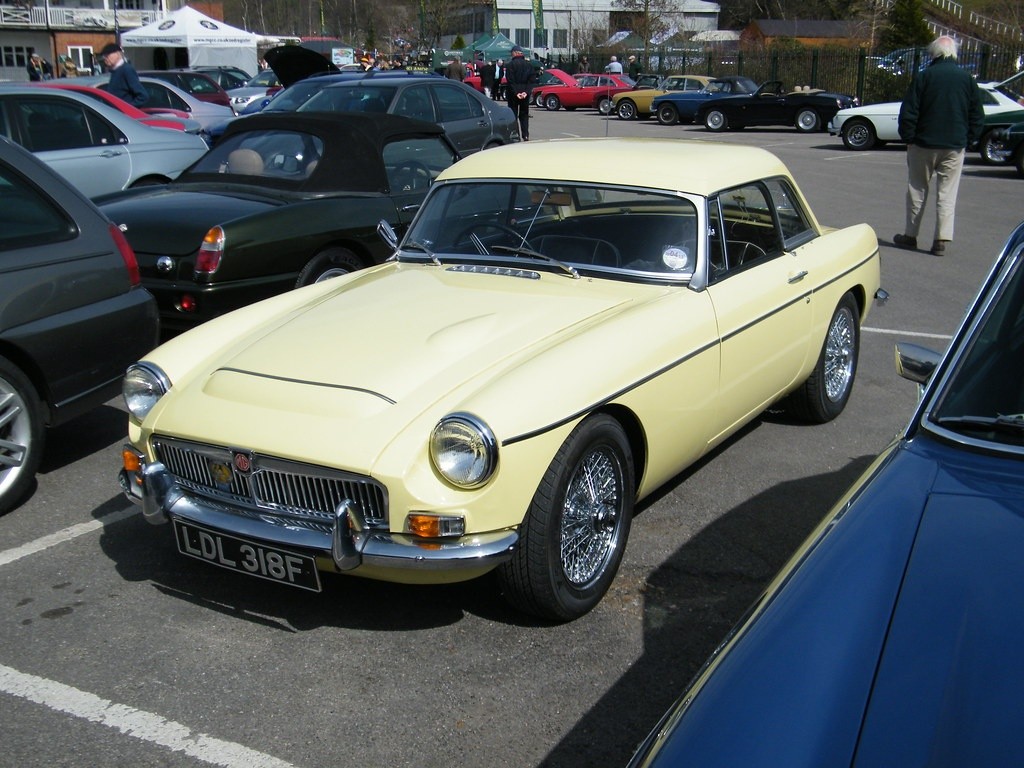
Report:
360,55,432,72
506,46,536,141
479,61,493,97
628,55,643,82
490,58,506,101
893,33,986,257
60,56,80,78
474,58,485,68
26,53,54,82
101,43,150,109
578,55,592,74
92,55,102,75
606,55,623,74
464,59,474,77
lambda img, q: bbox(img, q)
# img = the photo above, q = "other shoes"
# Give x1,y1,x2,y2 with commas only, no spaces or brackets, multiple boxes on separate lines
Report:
931,240,946,255
523,135,528,141
894,233,917,247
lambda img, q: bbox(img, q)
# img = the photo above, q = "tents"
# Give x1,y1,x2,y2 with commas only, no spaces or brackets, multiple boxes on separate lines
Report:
455,31,534,65
120,4,259,78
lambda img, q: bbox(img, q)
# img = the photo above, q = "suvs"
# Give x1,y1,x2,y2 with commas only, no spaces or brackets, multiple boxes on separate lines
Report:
196,70,449,148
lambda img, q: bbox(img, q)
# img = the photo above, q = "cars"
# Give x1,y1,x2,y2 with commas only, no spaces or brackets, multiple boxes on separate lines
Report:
90,112,604,345
828,70,1024,152
1,134,161,518
28,84,190,131
650,76,758,126
865,48,981,76
967,110,1024,166
540,69,636,111
43,76,236,130
0,86,209,199
136,45,361,116
591,76,659,116
694,81,859,134
239,79,520,169
609,74,730,121
116,135,892,625
625,225,1024,768
992,123,1024,179
462,68,591,108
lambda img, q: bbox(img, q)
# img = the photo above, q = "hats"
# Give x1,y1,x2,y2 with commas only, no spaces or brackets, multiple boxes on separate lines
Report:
628,55,635,59
101,44,120,56
32,53,39,57
508,46,522,52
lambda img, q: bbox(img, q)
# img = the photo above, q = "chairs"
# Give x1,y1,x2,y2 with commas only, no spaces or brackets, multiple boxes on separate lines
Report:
526,235,622,270
360,98,387,112
793,85,801,93
304,160,318,178
229,148,264,175
657,238,765,281
803,85,810,93
405,96,429,121
